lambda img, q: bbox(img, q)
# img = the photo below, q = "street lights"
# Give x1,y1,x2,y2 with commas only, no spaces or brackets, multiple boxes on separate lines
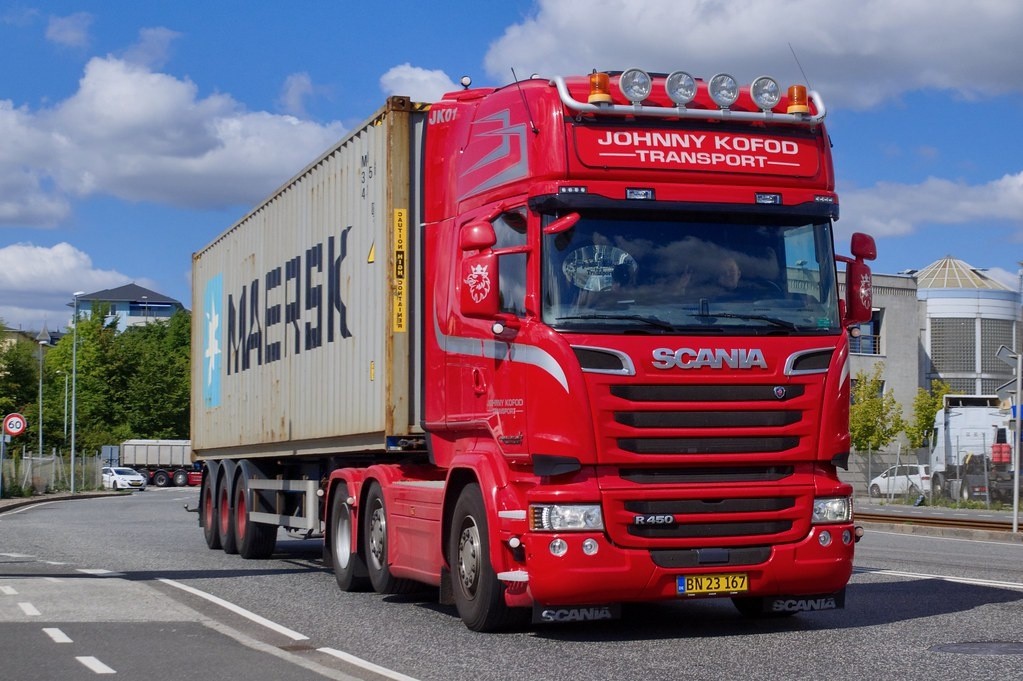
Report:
39,340,48,457
70,291,86,492
55,370,68,444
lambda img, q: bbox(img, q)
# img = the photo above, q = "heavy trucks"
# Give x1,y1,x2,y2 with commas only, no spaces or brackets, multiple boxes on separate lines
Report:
188,68,878,635
119,439,204,488
924,394,1023,505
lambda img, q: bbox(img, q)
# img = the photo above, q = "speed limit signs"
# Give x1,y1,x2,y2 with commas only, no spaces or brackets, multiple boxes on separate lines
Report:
4,413,27,436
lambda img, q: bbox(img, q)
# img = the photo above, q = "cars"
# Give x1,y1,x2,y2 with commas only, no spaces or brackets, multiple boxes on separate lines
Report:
100,466,147,492
867,464,931,500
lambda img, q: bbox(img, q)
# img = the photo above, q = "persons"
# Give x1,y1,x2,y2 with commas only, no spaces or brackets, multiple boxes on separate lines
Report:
611,255,769,318
